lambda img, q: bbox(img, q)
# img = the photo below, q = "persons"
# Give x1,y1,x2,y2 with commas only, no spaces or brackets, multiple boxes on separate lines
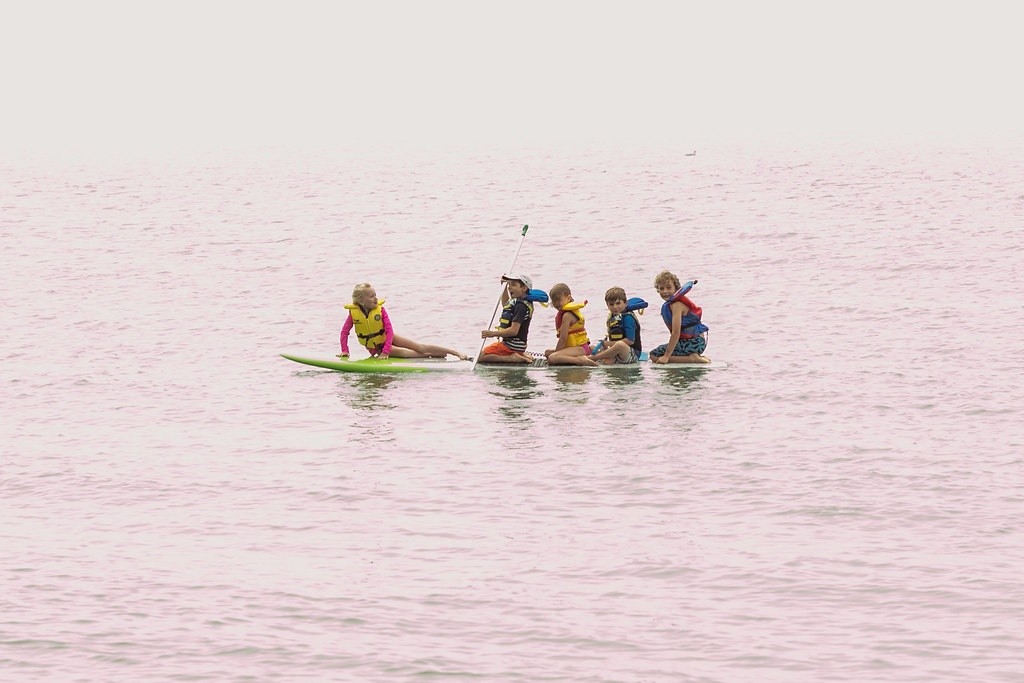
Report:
477,273,535,363
650,270,711,364
544,282,598,366
336,282,468,361
588,287,641,365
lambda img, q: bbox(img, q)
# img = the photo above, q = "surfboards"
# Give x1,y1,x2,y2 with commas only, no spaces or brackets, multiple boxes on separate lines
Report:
276,349,735,378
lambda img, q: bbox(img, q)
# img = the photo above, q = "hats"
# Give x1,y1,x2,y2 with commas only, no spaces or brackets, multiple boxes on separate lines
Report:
502,274,532,291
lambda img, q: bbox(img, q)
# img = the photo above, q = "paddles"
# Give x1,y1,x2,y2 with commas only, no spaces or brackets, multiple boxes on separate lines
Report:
472,225,529,371
